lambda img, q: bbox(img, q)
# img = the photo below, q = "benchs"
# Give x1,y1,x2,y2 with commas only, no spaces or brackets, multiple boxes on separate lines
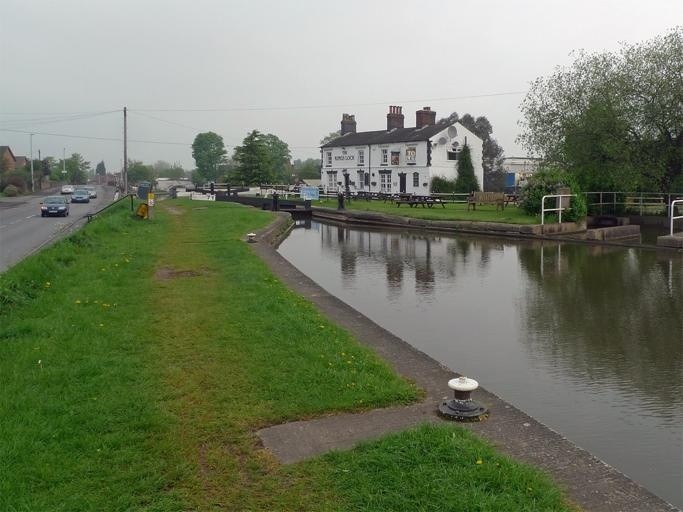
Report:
337,190,450,210
467,190,504,212
503,200,517,207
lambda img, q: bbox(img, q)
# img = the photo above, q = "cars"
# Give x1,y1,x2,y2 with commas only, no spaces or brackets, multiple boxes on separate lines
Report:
82,186,98,199
71,189,90,203
38,195,70,216
60,183,74,194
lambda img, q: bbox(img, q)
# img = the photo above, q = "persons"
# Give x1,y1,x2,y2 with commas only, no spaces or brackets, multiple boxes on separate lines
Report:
271,192,277,210
336,182,344,210
